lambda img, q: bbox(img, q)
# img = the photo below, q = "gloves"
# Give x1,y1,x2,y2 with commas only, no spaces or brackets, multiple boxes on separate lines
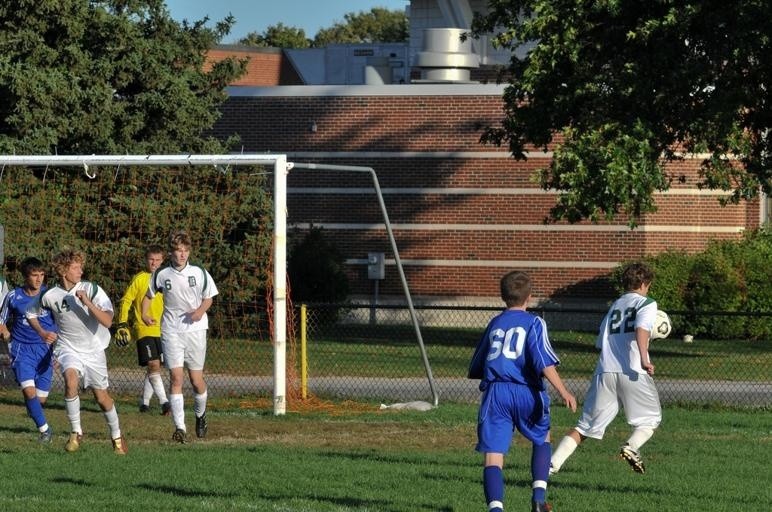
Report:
114,323,131,347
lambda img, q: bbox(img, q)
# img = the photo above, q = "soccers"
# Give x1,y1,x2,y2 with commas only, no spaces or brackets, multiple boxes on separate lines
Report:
651,311,671,341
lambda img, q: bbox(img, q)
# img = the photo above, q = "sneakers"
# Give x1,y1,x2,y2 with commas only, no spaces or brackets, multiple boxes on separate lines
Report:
620,442,645,474
26,402,207,454
532,502,552,512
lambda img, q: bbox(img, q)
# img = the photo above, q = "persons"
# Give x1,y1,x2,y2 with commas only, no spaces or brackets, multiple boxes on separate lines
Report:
141,233,218,443
546,263,663,477
114,244,171,417
466,271,577,511
0,256,58,443
25,249,128,457
